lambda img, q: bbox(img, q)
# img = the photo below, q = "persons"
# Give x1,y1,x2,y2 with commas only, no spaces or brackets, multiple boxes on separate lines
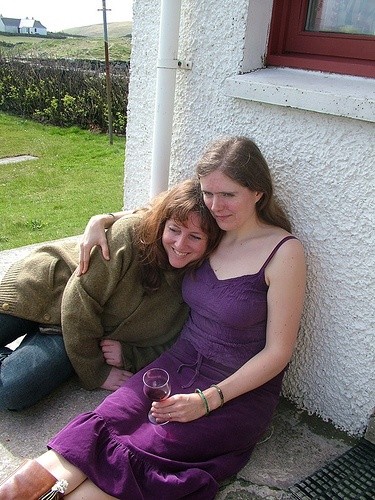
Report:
0,136,308,500
0,180,229,411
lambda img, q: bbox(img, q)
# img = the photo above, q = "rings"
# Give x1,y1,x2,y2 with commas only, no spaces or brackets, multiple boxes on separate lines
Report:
168,413,172,420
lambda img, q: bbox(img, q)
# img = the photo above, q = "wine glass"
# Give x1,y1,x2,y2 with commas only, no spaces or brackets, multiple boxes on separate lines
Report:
142,368,171,426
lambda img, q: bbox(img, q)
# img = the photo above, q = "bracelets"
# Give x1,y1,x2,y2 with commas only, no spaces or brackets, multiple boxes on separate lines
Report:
211,384,224,407
195,387,209,416
103,214,116,230
